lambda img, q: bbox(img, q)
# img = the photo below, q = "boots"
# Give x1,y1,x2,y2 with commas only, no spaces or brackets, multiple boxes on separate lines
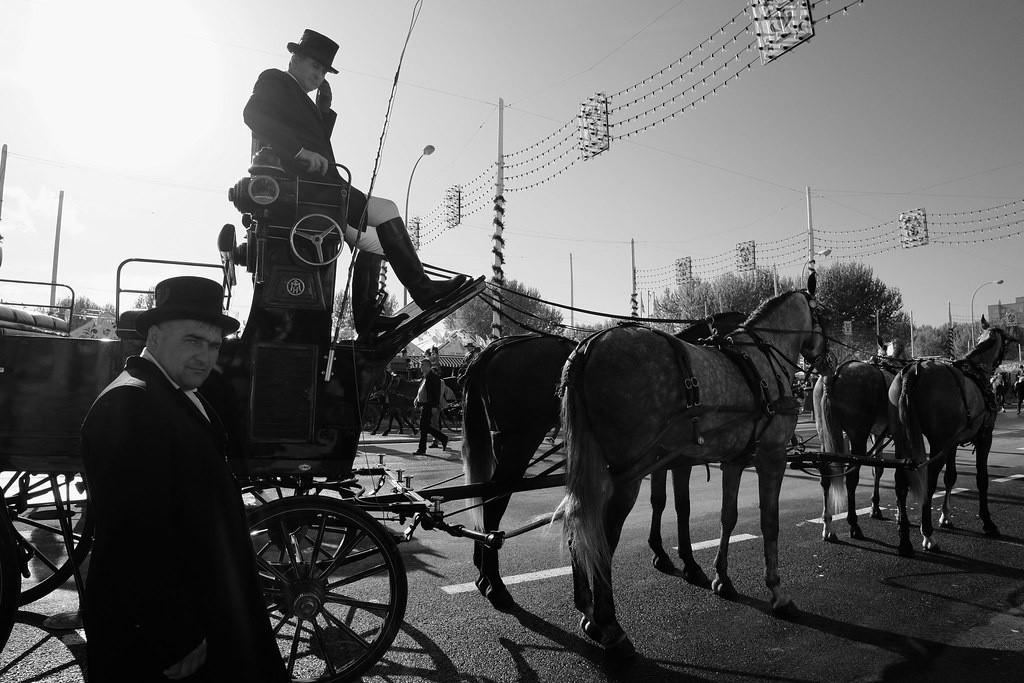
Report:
352,251,410,337
376,216,467,310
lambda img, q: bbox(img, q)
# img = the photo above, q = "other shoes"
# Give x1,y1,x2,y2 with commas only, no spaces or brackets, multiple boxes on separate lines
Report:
429,439,437,447
413,447,424,454
442,438,447,451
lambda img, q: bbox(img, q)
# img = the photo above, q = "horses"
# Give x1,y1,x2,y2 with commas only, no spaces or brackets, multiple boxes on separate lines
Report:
359,268,1024,668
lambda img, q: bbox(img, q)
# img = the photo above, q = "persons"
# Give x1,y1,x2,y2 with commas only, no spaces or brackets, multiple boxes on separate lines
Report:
412,343,481,456
75,276,293,683
792,373,818,422
991,364,1024,415
242,29,467,335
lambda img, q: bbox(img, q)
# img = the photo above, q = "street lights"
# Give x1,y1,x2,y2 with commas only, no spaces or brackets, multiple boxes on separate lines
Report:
400,145,438,357
971,279,1004,347
800,249,832,290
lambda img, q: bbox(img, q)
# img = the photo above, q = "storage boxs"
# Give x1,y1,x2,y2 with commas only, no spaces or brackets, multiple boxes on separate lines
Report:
248,341,319,446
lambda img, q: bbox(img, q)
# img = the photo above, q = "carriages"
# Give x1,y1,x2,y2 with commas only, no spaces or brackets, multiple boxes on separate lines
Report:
0,149,1024,683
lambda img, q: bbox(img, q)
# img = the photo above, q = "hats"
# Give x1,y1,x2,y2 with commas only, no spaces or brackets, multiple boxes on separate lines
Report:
134,276,241,339
286,29,340,73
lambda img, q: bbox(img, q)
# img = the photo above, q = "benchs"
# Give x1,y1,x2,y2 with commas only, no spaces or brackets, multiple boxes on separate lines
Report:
0,259,231,339
